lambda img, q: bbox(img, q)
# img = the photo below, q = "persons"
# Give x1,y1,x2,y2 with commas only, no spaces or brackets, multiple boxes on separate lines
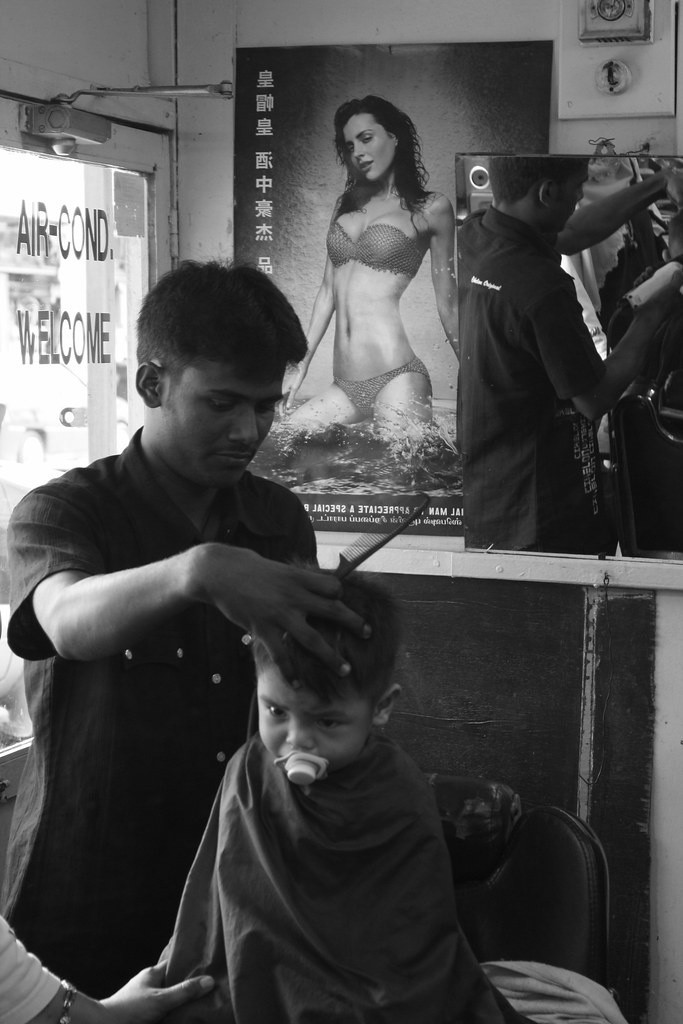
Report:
277,96,466,461
457,156,679,551
164,568,507,1024
1,262,315,1000
0,895,218,1024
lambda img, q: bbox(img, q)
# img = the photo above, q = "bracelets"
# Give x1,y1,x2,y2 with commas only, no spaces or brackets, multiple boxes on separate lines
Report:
59,980,78,1022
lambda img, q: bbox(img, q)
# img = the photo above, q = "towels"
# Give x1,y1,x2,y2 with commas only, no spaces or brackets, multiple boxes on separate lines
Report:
476,959,631,1024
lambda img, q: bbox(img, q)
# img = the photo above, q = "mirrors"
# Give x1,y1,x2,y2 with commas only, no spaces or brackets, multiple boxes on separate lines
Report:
454,151,683,563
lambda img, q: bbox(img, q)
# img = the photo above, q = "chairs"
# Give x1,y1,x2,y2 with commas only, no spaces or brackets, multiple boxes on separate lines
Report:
441,805,608,987
606,384,683,564
